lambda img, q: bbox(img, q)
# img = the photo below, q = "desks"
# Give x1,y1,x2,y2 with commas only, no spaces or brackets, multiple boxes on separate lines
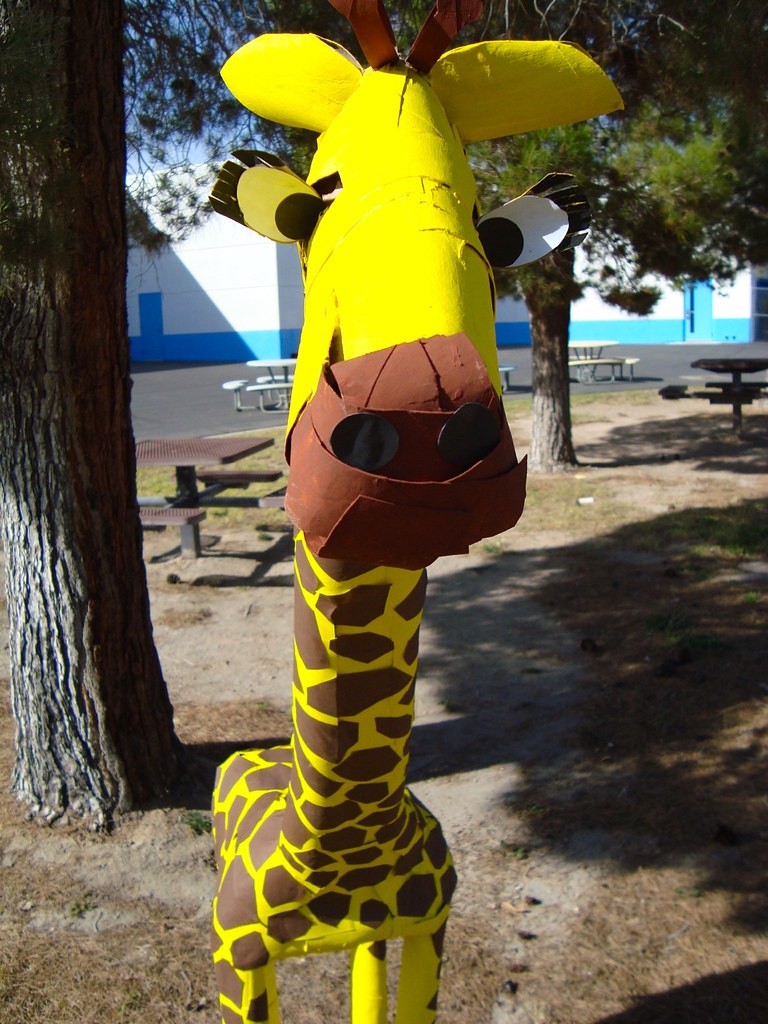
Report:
691,358,768,435
568,340,619,380
247,360,298,410
134,436,278,557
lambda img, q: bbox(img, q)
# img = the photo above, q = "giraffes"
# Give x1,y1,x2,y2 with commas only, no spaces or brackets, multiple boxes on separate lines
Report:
208,0,625,1024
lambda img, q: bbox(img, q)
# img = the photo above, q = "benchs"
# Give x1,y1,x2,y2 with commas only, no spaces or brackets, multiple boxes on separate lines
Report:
195,470,283,498
659,382,768,404
259,486,287,510
566,356,640,382
222,376,295,414
138,505,206,531
